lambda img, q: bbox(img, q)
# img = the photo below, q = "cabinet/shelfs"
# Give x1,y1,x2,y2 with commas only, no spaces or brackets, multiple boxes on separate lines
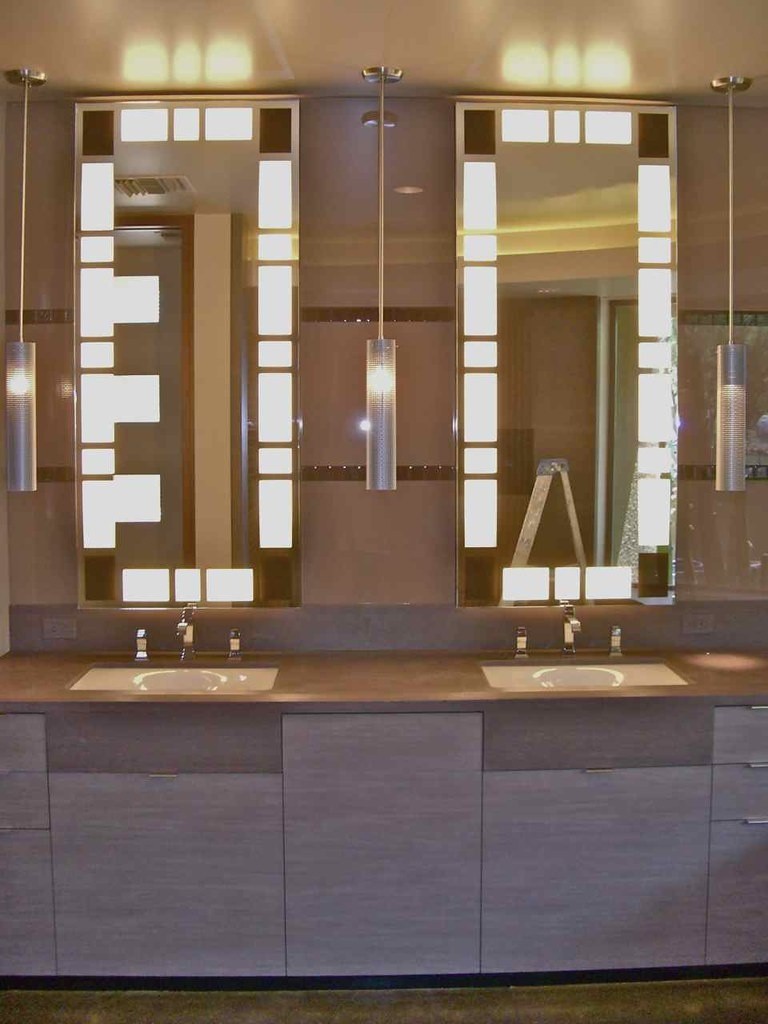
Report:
0,691,768,988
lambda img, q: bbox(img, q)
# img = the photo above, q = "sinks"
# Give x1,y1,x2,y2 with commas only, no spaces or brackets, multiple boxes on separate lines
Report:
62,660,280,693
478,656,695,691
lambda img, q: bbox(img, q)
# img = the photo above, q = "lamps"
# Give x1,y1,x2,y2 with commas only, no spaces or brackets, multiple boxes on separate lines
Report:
712,78,753,493
362,66,402,489
4,70,48,491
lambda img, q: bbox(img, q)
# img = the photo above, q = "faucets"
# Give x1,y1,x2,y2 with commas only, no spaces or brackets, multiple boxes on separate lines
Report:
557,602,582,655
170,605,197,663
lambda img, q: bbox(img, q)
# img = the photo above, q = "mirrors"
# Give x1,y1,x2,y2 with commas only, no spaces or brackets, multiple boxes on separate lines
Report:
456,101,676,607
75,102,304,609
